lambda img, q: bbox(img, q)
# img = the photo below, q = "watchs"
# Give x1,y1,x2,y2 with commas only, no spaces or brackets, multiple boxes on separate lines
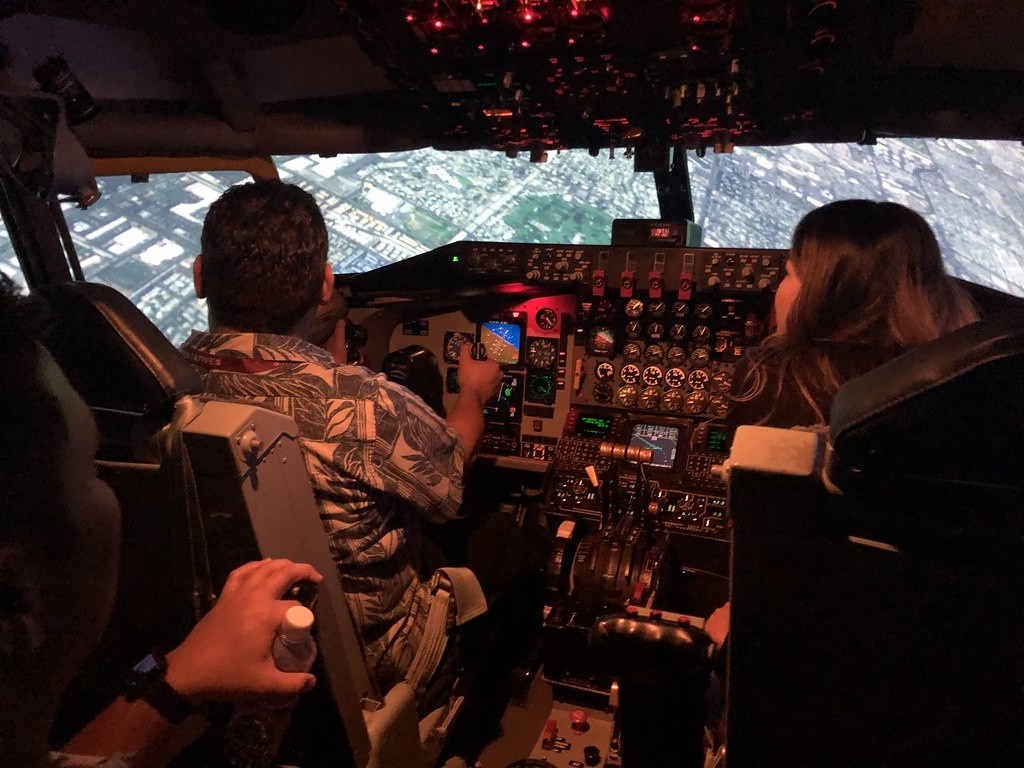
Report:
120,646,194,725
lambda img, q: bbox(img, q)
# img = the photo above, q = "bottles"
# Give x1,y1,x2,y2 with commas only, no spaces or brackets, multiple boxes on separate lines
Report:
221,606,316,764
2,45,101,207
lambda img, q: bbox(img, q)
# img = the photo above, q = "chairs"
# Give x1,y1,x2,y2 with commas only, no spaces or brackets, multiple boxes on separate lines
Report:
703,334,1024,768
31,277,467,768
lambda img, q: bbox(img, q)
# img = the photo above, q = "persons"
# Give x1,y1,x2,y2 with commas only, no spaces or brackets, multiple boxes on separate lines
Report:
180,179,519,762
0,272,324,768
703,199,980,768
